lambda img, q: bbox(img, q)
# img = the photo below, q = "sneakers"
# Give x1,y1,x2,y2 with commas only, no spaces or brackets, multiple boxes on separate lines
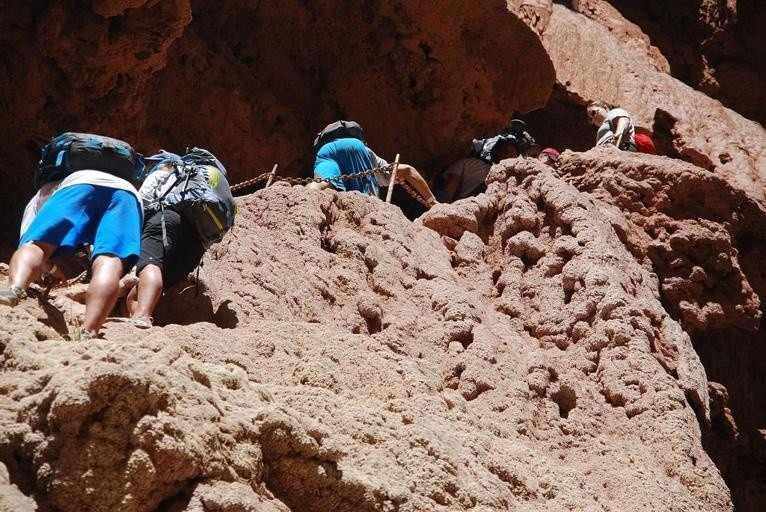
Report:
0,286,23,306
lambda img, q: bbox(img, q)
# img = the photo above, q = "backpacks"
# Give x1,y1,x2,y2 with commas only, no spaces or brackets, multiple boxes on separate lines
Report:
147,145,238,250
312,119,368,152
31,130,146,192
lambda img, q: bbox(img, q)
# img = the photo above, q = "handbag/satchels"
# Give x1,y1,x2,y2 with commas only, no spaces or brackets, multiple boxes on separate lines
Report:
634,133,656,155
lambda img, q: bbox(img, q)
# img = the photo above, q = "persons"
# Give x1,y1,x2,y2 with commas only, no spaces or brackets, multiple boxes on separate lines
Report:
304,137,438,210
123,148,207,328
443,155,494,204
537,147,559,169
0,166,143,342
586,99,639,153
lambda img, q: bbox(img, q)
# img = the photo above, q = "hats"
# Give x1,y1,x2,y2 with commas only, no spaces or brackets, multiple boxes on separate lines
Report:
542,148,560,163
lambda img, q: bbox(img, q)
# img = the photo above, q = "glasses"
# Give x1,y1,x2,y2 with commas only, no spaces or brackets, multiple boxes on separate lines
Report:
588,109,599,124
